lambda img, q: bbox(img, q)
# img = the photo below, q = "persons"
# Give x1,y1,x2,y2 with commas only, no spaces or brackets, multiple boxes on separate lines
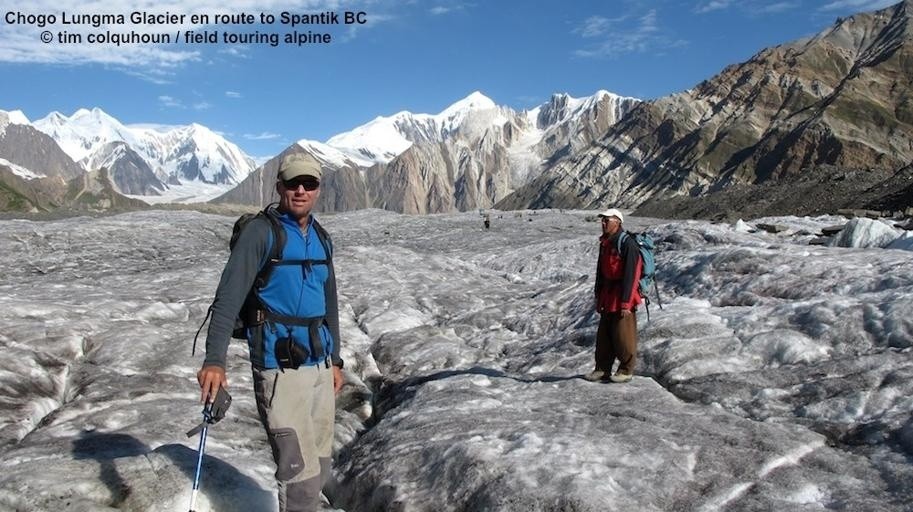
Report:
583,208,644,383
196,151,344,512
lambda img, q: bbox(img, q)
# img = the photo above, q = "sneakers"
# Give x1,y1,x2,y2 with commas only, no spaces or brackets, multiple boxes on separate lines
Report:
608,373,633,383
583,369,611,383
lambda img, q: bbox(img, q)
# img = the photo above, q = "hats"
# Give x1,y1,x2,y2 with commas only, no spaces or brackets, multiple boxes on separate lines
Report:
278,152,323,182
596,208,623,223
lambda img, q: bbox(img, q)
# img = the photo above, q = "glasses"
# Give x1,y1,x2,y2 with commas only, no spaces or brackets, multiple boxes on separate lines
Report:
283,178,320,192
601,218,619,223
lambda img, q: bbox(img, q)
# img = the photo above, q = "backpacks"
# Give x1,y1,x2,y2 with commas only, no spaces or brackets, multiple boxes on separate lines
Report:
230,203,330,339
618,230,664,298
